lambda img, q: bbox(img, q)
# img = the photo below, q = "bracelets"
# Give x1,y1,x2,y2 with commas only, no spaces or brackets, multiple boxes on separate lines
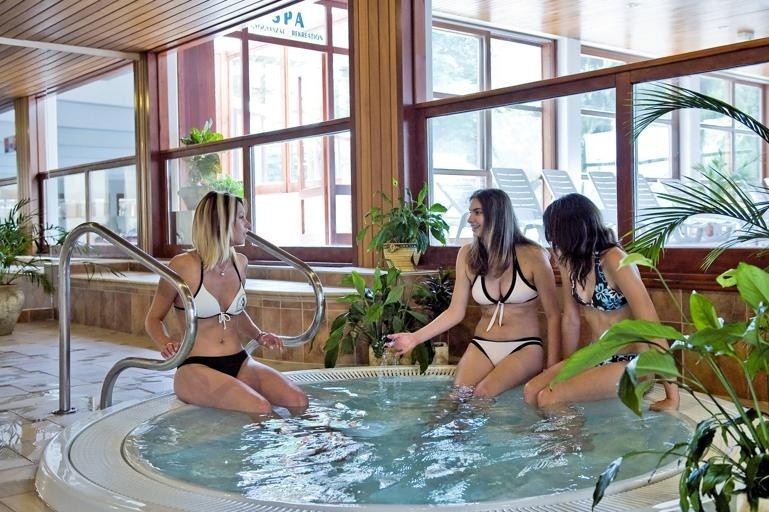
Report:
254,332,266,339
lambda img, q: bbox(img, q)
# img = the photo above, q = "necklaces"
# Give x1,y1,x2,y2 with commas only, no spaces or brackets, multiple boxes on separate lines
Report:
214,264,227,280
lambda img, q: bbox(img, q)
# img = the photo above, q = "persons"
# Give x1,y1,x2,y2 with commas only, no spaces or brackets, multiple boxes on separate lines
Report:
381,186,562,411
145,189,311,416
519,192,681,425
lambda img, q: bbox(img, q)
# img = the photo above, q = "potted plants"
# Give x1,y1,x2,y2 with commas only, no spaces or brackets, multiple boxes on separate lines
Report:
354,175,453,272
176,125,224,211
0,193,129,338
320,258,456,370
